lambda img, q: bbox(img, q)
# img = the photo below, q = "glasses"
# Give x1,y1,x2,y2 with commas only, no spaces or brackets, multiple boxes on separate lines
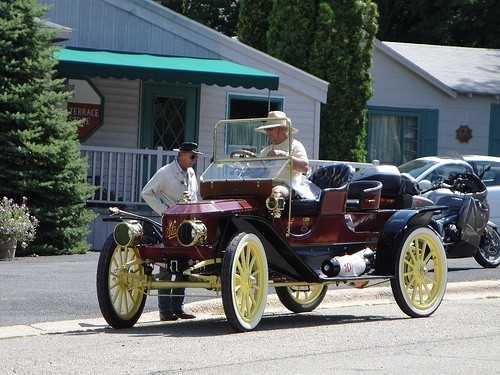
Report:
264,128,273,131
190,155,198,159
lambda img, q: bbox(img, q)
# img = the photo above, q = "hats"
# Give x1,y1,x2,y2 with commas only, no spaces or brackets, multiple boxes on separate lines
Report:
180,142,203,155
255,111,299,135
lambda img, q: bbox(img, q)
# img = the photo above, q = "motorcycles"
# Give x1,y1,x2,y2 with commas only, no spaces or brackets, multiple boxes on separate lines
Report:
350,162,500,268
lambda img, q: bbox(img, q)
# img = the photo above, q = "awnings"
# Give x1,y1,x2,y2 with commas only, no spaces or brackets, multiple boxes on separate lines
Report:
51,45,280,92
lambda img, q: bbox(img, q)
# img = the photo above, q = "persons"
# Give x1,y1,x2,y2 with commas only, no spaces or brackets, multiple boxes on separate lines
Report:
255,111,308,197
140,142,203,321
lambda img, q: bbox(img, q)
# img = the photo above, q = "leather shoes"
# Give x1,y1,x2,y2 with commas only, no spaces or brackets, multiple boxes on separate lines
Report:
159,311,179,321
171,310,196,319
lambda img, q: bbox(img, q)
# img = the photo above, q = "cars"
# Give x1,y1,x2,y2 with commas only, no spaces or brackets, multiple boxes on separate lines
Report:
395,154,500,231
96,118,449,334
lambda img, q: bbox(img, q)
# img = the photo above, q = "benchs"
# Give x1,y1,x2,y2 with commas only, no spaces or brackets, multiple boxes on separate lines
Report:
282,162,355,216
346,180,383,212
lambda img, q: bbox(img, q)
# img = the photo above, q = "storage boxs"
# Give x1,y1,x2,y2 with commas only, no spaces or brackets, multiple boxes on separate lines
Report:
349,165,402,199
